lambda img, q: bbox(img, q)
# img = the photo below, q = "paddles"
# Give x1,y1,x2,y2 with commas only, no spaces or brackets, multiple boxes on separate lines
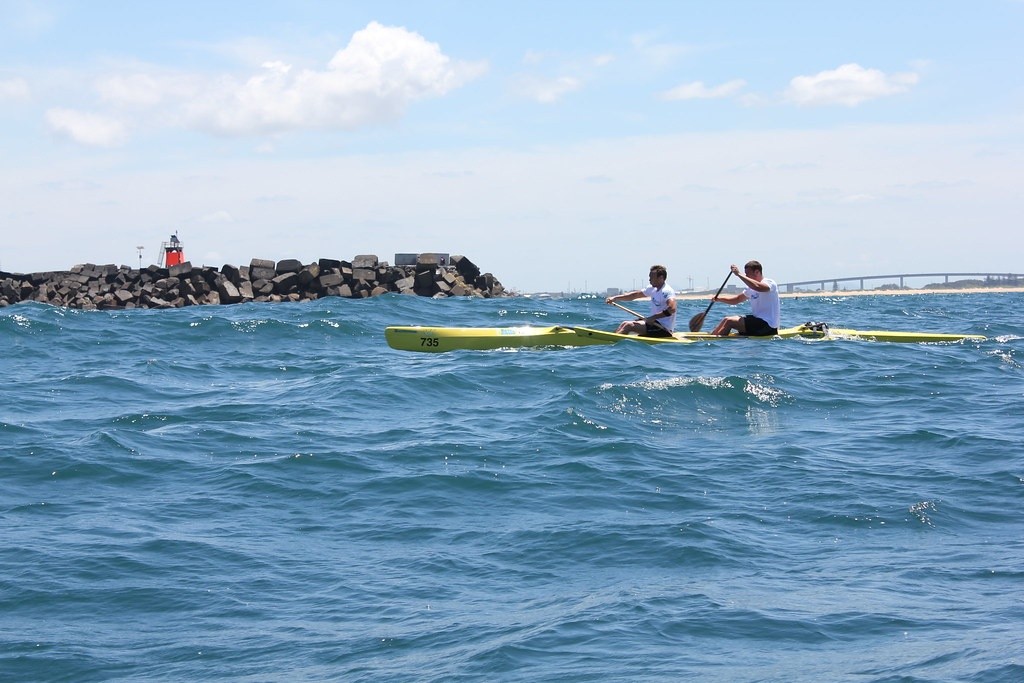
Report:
689,270,733,332
606,299,665,330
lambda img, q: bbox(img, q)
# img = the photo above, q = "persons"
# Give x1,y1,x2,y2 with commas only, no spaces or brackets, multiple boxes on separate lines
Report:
711,261,780,336
606,265,676,337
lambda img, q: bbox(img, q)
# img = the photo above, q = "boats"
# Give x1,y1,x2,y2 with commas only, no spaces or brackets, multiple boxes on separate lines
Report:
386,323,989,351
